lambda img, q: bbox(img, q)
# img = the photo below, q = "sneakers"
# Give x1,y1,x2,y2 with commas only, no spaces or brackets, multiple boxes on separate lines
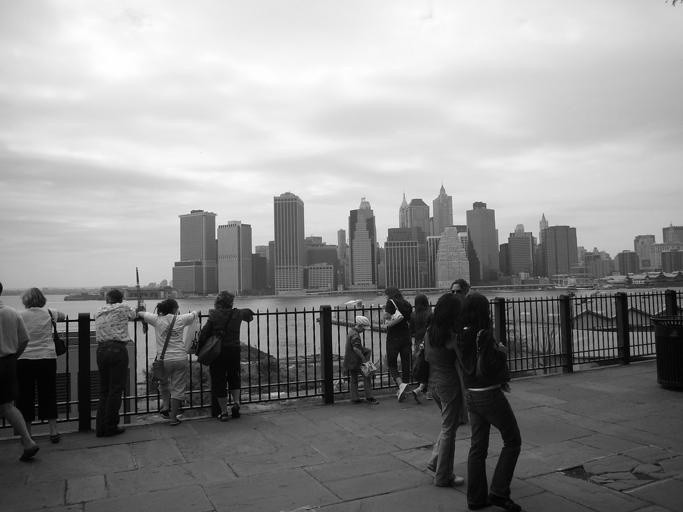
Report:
366,398,379,404
218,412,229,420
422,466,435,478
435,476,465,486
96,427,127,437
160,410,170,415
21,445,39,461
413,387,422,404
169,420,183,426
426,391,433,400
398,383,409,401
49,433,63,444
350,398,363,403
232,405,240,418
489,493,521,512
468,500,492,510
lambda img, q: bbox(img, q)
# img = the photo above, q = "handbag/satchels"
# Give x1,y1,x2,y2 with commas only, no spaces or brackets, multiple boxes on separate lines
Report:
48,309,66,357
384,299,404,330
464,328,507,386
197,308,236,366
360,361,377,380
150,315,177,392
413,328,428,382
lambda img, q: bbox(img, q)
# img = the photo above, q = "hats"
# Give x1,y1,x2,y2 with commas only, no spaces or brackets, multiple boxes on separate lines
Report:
356,316,371,330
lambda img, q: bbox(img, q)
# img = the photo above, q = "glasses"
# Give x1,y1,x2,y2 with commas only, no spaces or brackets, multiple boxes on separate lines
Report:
452,290,463,293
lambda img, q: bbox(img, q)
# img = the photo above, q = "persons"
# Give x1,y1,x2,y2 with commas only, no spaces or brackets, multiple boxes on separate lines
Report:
382,286,413,402
423,293,467,488
93,291,140,437
451,279,471,426
137,298,203,426
411,294,433,392
411,313,434,404
343,315,376,404
196,290,255,422
15,288,66,445
0,283,40,462
458,292,523,512
